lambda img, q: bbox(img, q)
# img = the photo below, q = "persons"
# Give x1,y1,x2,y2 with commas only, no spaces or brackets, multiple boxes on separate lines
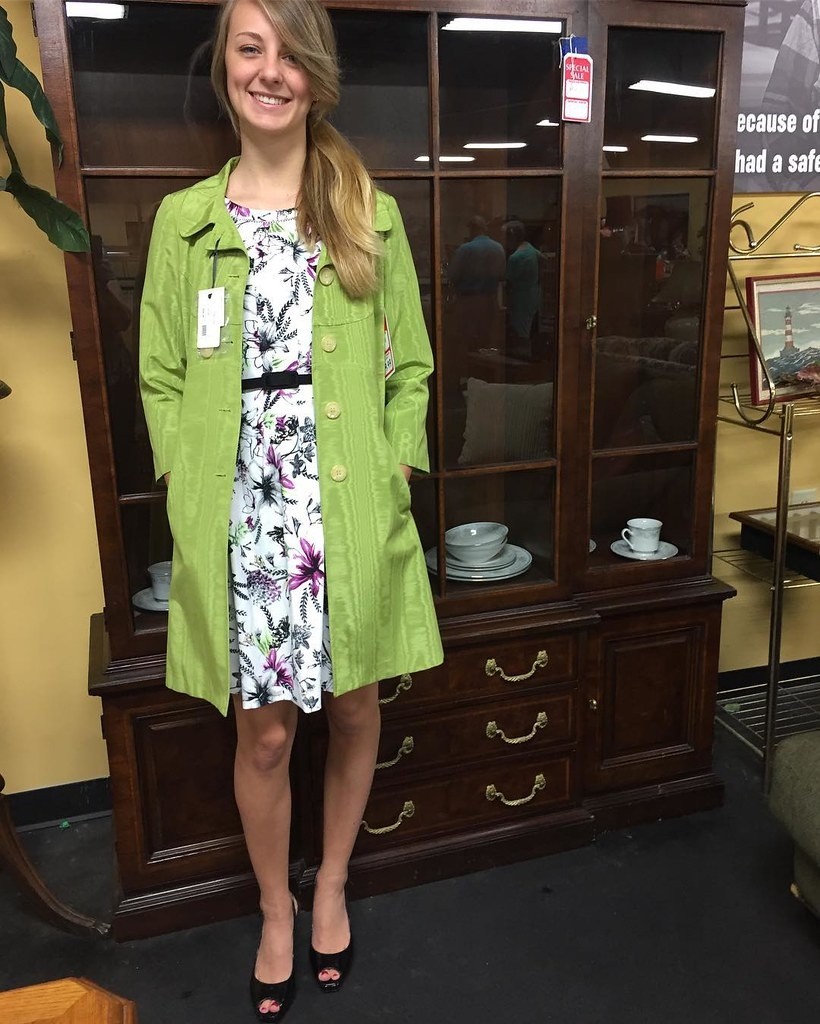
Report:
139,1,443,1024
450,214,506,341
501,220,541,358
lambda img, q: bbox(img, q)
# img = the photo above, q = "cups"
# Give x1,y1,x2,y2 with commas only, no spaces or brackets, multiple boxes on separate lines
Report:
621,518,663,556
148,562,172,600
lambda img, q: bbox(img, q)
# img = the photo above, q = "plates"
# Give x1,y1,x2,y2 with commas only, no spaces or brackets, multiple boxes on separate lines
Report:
132,587,169,611
425,543,533,581
610,539,678,560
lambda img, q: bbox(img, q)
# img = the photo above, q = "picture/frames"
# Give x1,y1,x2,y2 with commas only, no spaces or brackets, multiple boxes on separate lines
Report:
744,271,820,406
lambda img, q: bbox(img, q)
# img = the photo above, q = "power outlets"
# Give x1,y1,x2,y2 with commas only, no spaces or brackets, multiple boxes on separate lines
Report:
793,488,817,504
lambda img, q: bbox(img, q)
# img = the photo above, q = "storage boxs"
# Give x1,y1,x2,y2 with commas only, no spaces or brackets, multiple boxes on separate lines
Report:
728,501,819,581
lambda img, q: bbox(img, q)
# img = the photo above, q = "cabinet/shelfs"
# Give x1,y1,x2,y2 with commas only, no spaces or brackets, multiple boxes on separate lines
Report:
33,0,747,950
708,190,820,799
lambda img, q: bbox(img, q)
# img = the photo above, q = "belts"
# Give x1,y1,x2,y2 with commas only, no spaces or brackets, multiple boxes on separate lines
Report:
241,371,312,389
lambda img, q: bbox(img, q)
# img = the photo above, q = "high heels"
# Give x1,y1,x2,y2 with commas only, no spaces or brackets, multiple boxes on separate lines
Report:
249,895,300,1024
309,879,353,993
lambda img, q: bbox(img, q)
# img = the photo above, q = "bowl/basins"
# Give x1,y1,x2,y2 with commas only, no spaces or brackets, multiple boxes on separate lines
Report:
445,522,509,563
